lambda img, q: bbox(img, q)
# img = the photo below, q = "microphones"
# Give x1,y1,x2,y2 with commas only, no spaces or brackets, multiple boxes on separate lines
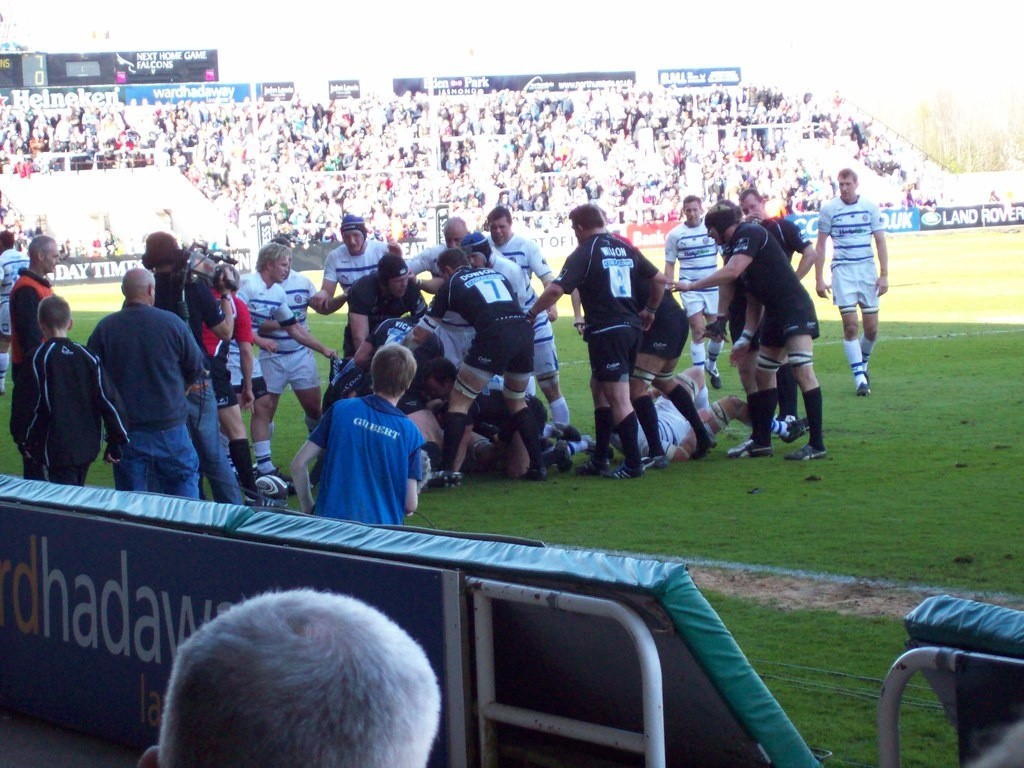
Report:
207,253,239,265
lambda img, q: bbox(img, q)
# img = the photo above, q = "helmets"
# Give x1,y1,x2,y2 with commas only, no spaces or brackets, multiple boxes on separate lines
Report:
705,200,742,234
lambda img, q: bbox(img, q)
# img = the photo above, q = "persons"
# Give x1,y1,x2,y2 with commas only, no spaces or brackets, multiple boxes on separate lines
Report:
137,588,441,768
816,168,887,396
290,344,427,528
120,188,826,510
0,230,31,395
9,236,60,482
17,294,129,486
19,83,939,272
85,267,205,500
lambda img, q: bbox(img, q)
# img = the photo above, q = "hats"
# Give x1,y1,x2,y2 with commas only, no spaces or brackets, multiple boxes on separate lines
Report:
340,214,367,239
460,231,490,262
378,255,407,280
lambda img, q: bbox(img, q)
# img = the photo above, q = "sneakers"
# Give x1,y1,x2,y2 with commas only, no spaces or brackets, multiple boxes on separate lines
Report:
579,434,614,461
603,462,643,480
228,458,242,484
428,469,463,488
704,359,721,389
691,433,718,459
780,418,811,443
256,464,297,494
784,444,827,460
646,457,669,468
550,422,581,442
854,371,870,396
727,439,774,459
575,459,610,475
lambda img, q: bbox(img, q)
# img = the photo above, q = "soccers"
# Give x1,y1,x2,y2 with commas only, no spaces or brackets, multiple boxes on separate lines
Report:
255,475,289,500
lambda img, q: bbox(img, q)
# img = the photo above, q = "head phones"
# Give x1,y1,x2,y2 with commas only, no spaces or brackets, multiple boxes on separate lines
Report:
141,252,155,270
